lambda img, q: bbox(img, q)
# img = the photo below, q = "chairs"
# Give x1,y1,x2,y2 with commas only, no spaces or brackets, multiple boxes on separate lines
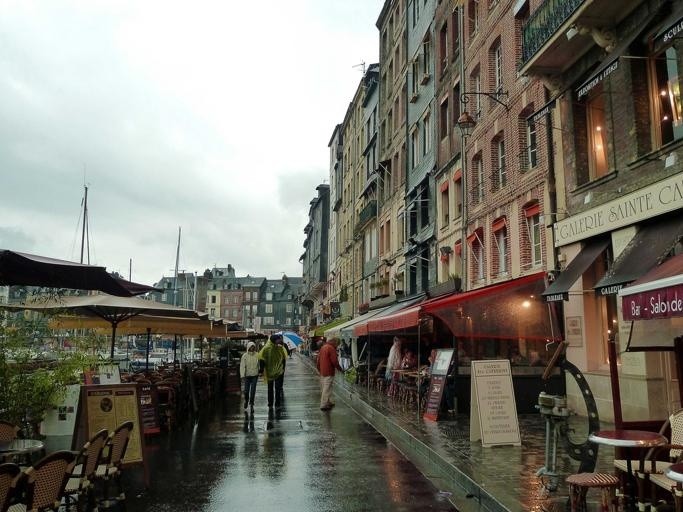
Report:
0,418,134,512
613,407,682,512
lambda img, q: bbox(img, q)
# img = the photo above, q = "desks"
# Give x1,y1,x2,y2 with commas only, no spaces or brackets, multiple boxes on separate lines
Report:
588,429,669,511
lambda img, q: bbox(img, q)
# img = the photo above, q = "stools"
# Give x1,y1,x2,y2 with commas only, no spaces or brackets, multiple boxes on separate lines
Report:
565,473,619,512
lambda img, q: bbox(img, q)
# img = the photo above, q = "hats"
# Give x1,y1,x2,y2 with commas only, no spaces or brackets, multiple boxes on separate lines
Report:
270,335,281,343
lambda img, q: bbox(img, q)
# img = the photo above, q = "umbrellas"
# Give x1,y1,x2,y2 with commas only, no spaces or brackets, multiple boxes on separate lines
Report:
1,251,253,374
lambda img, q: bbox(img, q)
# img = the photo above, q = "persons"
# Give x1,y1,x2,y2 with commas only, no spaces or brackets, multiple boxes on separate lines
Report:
428,350,436,370
257,331,305,411
316,335,345,411
384,334,417,399
239,341,260,412
337,337,353,372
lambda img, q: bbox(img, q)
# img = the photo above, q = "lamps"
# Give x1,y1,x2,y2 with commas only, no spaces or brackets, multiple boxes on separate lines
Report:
456,90,509,137
665,152,680,168
584,192,619,204
538,212,569,225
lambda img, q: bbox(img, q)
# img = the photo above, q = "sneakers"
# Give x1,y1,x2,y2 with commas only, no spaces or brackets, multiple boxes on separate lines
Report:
268,402,280,406
321,403,335,411
244,402,254,408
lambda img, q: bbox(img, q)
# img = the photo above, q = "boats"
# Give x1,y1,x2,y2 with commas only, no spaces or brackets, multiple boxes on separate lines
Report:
64,187,250,371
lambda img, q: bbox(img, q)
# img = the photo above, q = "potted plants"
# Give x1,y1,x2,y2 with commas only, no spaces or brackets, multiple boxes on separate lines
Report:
429,273,461,298
